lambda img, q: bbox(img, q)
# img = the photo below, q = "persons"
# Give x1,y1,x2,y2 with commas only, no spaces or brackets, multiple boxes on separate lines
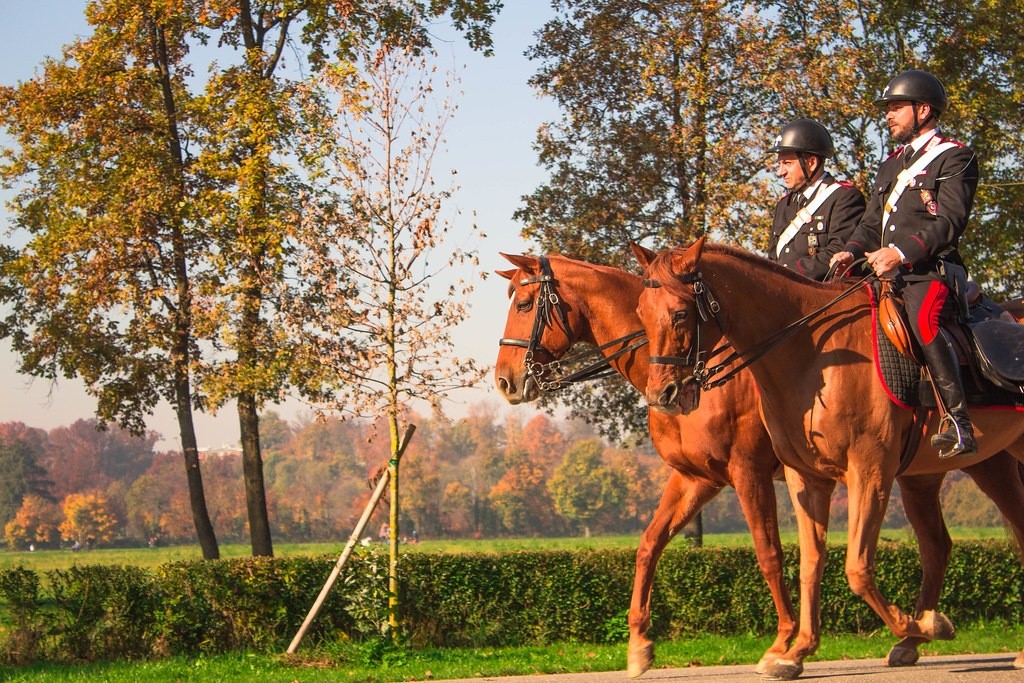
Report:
763,119,866,282
829,71,978,455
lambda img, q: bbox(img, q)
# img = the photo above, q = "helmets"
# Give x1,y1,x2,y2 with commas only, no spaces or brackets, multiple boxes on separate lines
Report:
873,68,947,114
765,119,834,160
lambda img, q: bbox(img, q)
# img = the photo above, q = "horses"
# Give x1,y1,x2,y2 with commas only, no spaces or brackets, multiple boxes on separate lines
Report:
626,235,1024,676
493,248,1024,679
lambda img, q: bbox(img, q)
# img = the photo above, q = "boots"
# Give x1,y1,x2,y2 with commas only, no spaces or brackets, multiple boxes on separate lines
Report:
921,328,978,454
